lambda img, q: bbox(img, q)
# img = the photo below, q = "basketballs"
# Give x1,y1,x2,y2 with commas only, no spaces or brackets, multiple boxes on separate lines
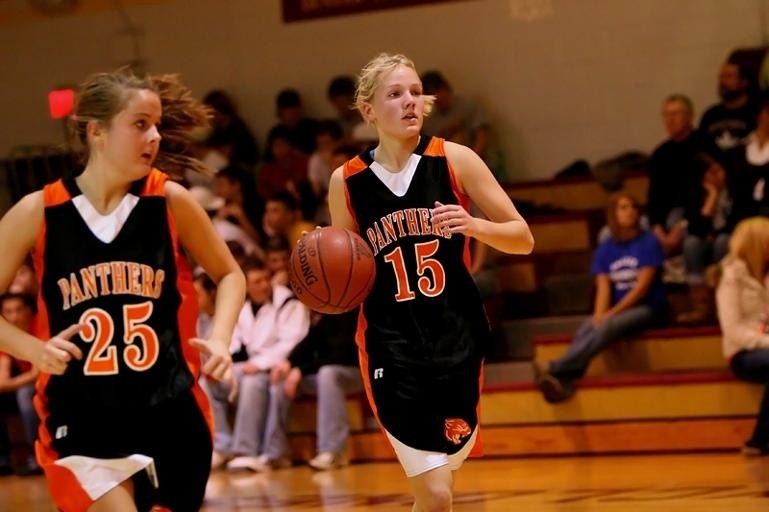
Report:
287,225,376,315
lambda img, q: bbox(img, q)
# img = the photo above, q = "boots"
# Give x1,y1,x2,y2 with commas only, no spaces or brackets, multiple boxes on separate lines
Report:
675,281,713,328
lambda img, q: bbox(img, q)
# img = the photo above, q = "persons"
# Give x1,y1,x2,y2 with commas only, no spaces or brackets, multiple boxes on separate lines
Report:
210,161,261,248
422,68,511,184
715,217,769,458
7,264,35,294
326,70,368,140
316,143,367,226
195,90,262,167
296,53,536,512
648,93,722,286
257,125,310,206
0,296,43,477
675,157,737,327
182,271,217,377
258,193,304,257
540,191,674,403
304,115,350,199
267,86,326,154
226,239,247,267
697,44,762,159
469,203,493,298
2,65,247,512
198,255,310,472
263,241,294,277
260,300,366,473
725,99,768,233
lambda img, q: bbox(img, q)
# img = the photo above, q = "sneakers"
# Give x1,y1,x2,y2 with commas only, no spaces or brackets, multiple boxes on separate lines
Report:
308,452,350,470
244,456,292,473
226,455,253,473
742,444,762,456
539,376,570,403
